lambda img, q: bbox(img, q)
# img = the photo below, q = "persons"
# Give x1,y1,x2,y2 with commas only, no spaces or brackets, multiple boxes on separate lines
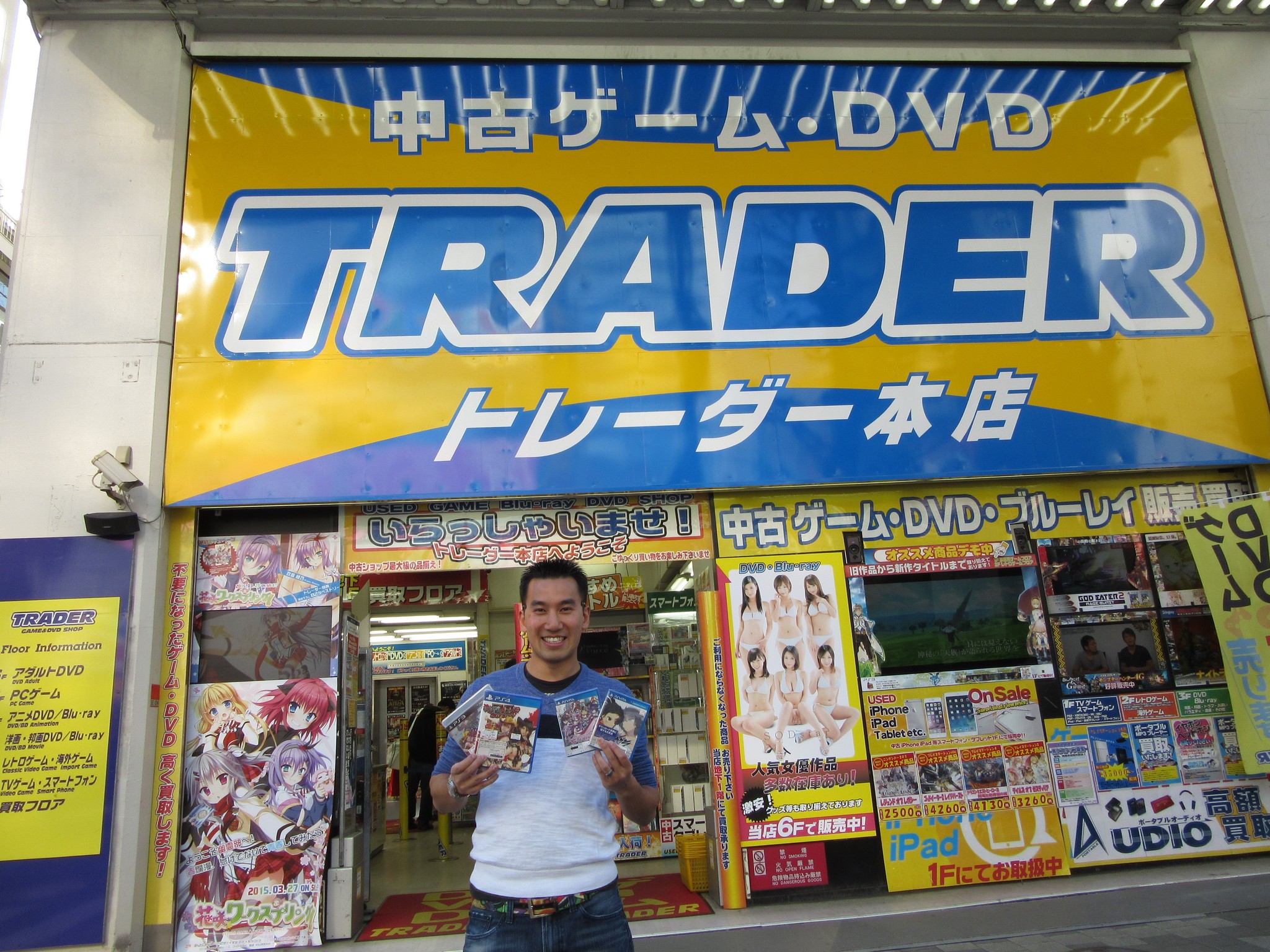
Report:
408,696,459,829
429,559,663,952
1117,628,1154,672
730,574,859,761
1071,634,1110,675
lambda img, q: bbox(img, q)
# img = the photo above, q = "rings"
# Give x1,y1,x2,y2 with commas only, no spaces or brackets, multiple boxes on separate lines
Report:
605,768,614,776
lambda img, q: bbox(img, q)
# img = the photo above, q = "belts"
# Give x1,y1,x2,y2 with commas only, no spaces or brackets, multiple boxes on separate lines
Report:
473,885,611,916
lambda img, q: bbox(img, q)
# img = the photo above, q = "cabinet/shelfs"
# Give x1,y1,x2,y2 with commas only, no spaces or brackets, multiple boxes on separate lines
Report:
607,675,663,861
646,589,714,858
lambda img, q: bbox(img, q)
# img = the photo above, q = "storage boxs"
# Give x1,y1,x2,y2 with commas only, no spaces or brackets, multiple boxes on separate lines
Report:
654,644,713,814
704,805,724,906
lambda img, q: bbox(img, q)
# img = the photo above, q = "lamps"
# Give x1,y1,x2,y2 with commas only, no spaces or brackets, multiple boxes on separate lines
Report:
662,560,694,591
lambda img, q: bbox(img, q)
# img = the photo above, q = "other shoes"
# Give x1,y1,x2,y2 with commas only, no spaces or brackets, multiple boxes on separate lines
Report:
408,822,417,829
418,823,434,830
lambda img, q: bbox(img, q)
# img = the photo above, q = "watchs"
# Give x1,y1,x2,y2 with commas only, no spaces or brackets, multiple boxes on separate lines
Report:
446,774,470,799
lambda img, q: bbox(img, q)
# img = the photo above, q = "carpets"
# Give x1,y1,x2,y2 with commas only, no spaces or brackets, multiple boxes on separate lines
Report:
354,873,716,942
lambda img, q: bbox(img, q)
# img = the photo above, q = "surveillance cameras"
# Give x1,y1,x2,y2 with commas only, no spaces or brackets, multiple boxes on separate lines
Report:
91,451,144,508
